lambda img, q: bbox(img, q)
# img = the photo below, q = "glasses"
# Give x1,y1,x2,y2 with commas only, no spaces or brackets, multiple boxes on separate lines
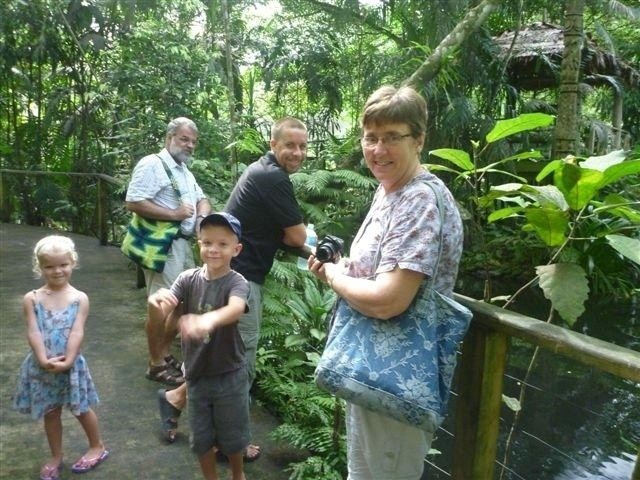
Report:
174,133,199,147
359,133,413,150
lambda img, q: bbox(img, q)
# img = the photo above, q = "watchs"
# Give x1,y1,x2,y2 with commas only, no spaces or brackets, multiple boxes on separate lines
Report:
194,214,208,218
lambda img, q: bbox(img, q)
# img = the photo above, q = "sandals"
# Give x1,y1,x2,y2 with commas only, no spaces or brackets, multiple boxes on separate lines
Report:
164,354,183,373
39,453,64,480
145,359,185,386
72,450,110,473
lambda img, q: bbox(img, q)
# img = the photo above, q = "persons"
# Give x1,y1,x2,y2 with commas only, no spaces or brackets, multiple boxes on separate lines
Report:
149,211,252,480
306,86,464,480
12,235,109,480
157,118,307,462
126,117,211,385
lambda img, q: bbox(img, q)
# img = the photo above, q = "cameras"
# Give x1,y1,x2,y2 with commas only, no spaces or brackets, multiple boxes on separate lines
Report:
315,235,345,265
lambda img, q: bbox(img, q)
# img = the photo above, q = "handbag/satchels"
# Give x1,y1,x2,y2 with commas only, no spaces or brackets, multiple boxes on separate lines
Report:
120,212,183,273
314,275,473,435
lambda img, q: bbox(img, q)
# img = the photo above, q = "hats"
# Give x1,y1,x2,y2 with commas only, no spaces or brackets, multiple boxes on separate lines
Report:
200,212,242,243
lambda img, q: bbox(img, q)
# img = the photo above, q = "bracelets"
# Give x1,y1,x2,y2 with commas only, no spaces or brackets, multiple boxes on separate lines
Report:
327,278,333,291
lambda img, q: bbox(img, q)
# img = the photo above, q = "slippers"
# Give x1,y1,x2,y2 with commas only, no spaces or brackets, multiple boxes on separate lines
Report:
157,388,182,443
215,442,263,463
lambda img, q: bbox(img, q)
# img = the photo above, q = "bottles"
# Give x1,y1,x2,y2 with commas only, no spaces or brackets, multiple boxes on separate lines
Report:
297,223,319,271
